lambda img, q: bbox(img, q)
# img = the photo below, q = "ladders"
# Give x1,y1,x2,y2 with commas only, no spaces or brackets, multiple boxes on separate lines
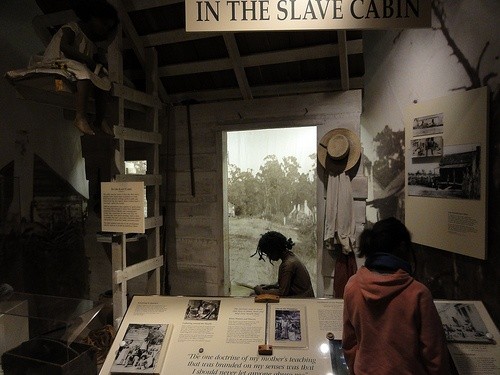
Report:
107,23,164,333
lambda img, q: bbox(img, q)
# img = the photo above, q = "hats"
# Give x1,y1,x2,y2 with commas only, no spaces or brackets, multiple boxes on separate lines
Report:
318,128,361,173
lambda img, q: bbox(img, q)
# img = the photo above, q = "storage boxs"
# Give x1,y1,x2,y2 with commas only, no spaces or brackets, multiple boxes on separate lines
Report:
6,336,98,375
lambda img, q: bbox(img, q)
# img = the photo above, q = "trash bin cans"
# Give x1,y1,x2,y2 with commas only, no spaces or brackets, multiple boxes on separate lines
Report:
2,337,97,375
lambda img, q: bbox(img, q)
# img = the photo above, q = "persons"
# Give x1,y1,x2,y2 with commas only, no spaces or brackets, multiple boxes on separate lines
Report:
425,138,438,156
442,316,477,339
408,169,432,185
467,168,480,200
184,300,206,320
276,313,301,341
42,0,120,137
341,218,460,375
421,119,436,127
250,230,315,298
115,327,165,370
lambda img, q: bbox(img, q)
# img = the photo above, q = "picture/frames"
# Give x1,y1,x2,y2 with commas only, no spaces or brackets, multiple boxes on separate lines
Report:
270,304,307,347
